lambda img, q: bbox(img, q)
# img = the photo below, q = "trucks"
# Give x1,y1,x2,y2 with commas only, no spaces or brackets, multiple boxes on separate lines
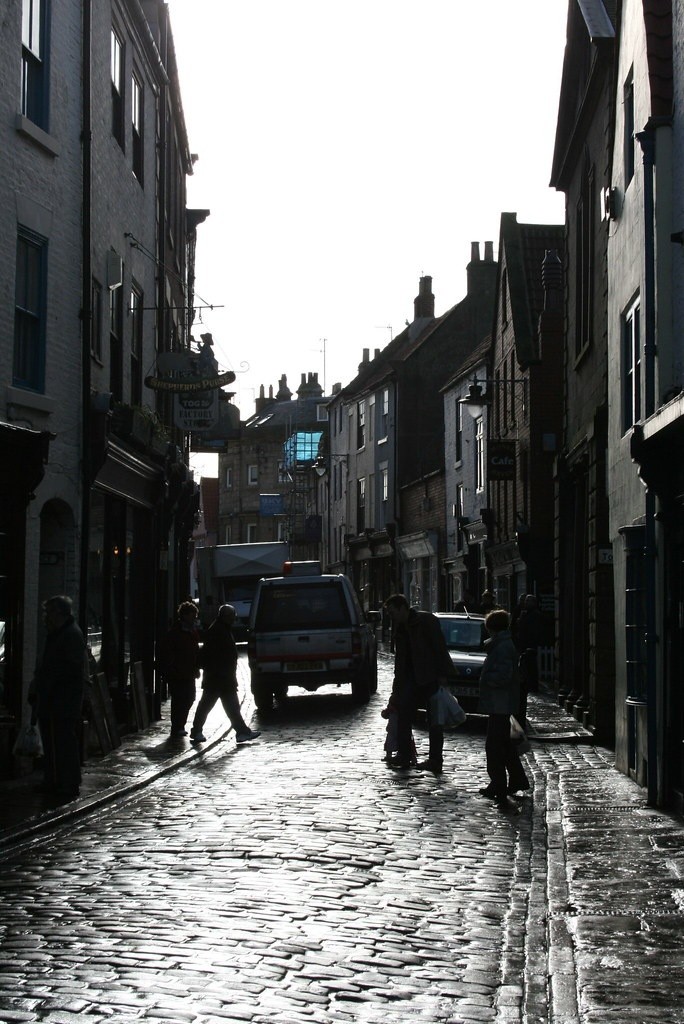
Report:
195,541,292,642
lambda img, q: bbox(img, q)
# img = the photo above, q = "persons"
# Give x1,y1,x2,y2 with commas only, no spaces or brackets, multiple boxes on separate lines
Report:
453,600,472,644
381,594,455,774
164,601,200,737
28,595,84,797
478,592,540,686
190,604,261,742
478,609,530,798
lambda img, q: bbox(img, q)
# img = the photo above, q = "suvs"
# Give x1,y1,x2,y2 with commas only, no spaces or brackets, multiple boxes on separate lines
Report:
242,558,383,709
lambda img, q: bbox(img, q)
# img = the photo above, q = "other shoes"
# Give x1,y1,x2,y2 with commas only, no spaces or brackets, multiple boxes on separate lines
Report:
479,779,506,798
391,750,416,765
507,774,530,794
236,730,262,742
189,728,207,741
416,759,444,774
170,727,188,735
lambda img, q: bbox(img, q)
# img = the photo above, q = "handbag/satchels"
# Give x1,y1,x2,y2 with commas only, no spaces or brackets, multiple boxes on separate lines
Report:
442,689,466,730
12,726,44,758
507,716,531,758
428,694,444,727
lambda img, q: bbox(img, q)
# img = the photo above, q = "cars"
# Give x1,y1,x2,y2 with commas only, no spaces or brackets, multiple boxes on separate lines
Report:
417,609,536,721
